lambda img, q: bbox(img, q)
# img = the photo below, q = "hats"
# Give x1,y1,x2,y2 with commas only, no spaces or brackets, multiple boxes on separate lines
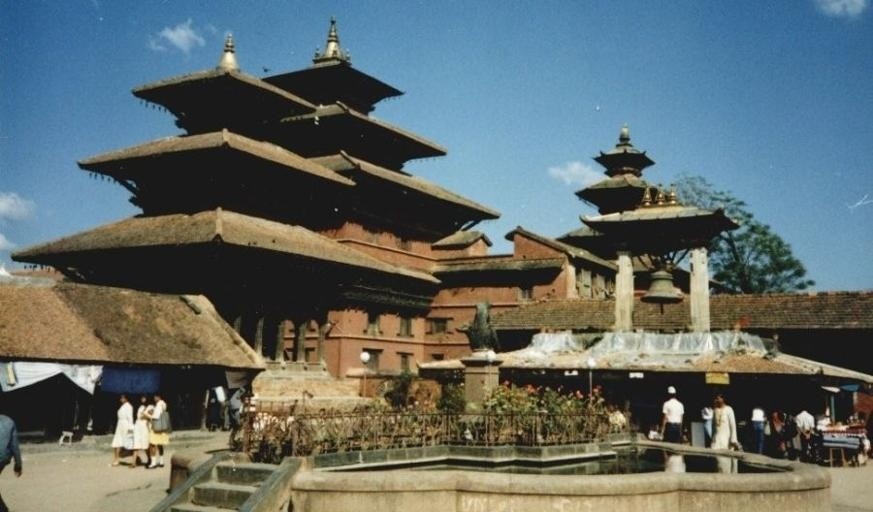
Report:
668,385,678,393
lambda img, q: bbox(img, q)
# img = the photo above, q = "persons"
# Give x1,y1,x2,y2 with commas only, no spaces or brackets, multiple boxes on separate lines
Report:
751,408,815,463
112,391,170,469
204,379,246,449
0,414,21,512
660,386,684,442
701,394,738,450
662,454,738,472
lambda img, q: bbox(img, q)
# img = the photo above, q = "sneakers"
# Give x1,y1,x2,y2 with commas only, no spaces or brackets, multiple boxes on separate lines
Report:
112,460,166,469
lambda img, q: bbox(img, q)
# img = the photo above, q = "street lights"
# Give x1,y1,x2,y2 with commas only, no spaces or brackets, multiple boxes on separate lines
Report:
483,350,496,390
359,351,371,404
586,356,595,402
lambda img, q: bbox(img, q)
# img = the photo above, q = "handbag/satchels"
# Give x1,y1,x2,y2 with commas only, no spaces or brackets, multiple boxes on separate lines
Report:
152,410,171,433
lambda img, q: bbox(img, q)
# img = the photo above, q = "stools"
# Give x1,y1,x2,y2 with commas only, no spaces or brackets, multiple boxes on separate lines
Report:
58,431,72,447
